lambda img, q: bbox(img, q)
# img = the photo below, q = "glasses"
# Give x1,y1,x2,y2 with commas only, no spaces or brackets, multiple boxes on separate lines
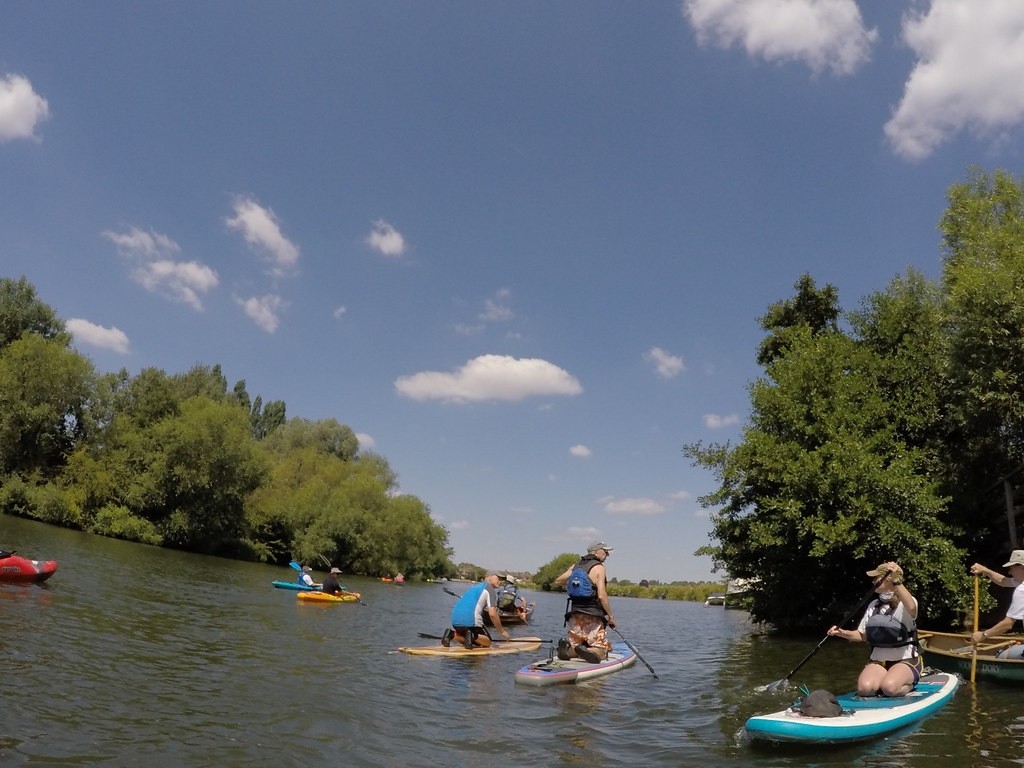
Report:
336,573,342,575
604,550,610,554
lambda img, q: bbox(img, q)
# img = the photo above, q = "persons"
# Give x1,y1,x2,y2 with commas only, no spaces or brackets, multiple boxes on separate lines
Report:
395,573,403,581
970,550,1024,660
386,573,391,579
495,575,526,620
323,568,357,596
555,541,617,664
827,561,923,697
441,570,510,649
297,566,322,587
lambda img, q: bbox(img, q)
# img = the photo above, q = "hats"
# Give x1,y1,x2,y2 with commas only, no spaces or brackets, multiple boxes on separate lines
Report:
1002,550,1024,567
330,567,342,574
507,575,515,583
484,569,507,581
586,541,615,551
867,564,904,578
301,565,312,572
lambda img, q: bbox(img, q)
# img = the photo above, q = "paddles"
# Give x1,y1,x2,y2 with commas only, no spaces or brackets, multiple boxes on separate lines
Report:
417,631,554,644
289,561,302,573
610,624,658,678
442,585,462,598
354,594,367,607
763,568,893,690
969,569,980,684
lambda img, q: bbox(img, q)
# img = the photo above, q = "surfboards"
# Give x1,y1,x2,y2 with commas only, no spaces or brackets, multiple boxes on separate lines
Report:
396,635,542,657
743,671,962,745
515,641,638,687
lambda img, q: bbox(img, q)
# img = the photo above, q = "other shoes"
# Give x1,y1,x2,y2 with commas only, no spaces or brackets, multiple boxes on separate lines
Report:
557,638,571,660
466,630,473,648
442,628,454,647
574,645,601,664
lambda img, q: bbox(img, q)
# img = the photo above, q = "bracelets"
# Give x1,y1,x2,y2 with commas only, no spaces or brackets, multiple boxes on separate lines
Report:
982,632,988,638
893,577,902,586
